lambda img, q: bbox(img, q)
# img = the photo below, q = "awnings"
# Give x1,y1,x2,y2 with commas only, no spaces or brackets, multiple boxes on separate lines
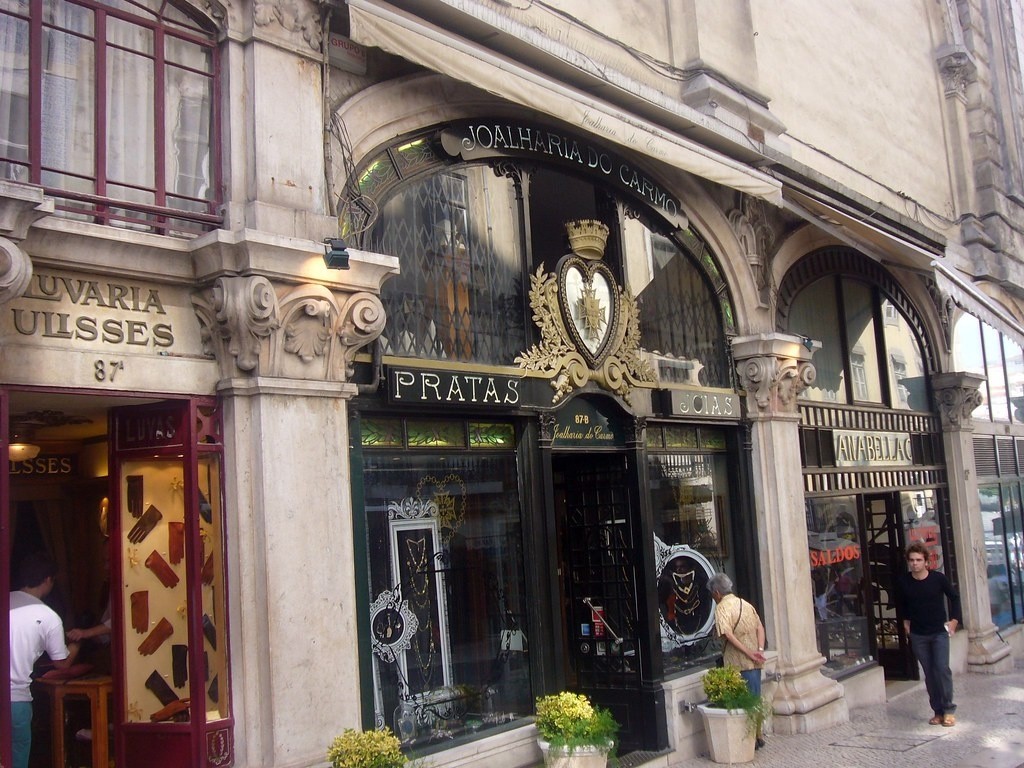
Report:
784,189,1024,349
345,0,783,209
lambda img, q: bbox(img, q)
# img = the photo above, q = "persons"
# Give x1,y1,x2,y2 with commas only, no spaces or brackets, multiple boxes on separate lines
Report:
706,573,767,750
9,556,82,768
896,542,962,727
66,539,112,646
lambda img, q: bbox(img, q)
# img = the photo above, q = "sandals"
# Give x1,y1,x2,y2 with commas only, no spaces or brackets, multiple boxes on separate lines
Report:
929,715,943,724
942,714,956,726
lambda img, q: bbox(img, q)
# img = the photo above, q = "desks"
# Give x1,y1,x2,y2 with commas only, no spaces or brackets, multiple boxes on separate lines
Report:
37,672,116,768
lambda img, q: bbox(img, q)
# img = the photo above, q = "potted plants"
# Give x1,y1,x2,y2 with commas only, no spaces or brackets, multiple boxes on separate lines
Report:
698,663,770,764
535,690,621,768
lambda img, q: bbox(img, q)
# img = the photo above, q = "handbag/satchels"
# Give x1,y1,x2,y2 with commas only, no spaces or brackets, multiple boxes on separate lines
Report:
715,597,742,668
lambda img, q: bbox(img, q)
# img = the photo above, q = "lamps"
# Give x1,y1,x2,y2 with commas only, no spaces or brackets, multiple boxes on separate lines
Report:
801,334,813,352
8,414,42,462
322,237,350,270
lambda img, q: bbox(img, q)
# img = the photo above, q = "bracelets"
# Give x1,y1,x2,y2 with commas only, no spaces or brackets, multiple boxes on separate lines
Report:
759,648,764,651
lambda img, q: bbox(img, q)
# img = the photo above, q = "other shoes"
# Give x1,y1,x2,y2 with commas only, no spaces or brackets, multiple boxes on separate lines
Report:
755,738,765,750
